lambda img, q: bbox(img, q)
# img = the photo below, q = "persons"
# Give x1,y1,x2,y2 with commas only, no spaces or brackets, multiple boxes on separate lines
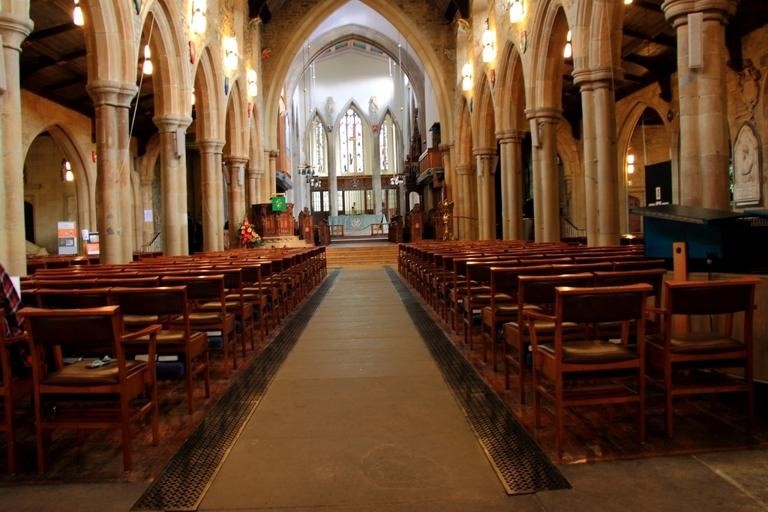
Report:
0,264,83,434
224,220,230,249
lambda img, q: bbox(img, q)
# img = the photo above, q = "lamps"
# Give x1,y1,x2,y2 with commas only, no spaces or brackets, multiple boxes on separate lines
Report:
310,176,322,188
72,0,85,26
461,0,522,91
141,44,153,76
563,29,572,58
192,0,258,100
390,174,404,185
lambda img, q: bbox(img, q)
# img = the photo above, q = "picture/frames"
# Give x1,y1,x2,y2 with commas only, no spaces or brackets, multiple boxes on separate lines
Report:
731,119,762,207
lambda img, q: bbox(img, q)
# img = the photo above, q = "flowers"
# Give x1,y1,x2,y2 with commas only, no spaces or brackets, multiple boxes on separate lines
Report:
238,216,262,244
